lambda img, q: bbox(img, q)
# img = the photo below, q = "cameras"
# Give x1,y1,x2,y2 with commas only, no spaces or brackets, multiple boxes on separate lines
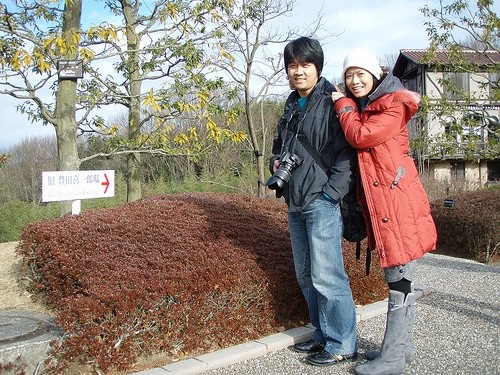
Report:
264,151,304,198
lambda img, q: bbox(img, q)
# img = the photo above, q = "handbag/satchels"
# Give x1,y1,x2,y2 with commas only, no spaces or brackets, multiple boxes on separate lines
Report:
340,192,368,242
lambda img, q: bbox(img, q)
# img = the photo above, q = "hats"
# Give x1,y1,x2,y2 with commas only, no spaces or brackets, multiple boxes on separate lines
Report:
341,48,383,87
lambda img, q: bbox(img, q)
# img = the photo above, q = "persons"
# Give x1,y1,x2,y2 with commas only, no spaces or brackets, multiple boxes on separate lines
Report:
268,35,359,367
330,51,438,375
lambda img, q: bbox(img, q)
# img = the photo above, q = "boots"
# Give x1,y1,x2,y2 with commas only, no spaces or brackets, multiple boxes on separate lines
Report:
366,283,415,362
354,289,414,375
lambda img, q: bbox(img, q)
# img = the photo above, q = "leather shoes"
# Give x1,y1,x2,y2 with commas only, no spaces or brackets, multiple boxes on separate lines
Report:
294,339,326,353
307,350,357,367
